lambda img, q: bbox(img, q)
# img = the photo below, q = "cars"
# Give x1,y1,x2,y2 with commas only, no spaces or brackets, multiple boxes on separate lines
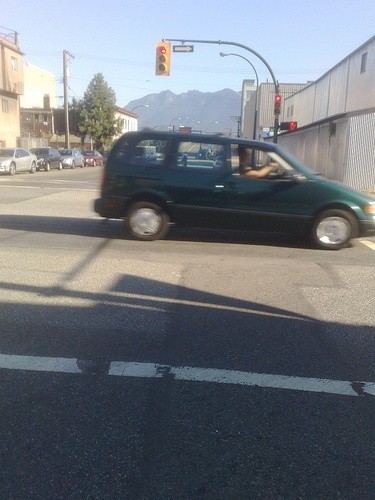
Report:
81,150,104,168
0,147,38,175
28,146,64,172
58,148,86,169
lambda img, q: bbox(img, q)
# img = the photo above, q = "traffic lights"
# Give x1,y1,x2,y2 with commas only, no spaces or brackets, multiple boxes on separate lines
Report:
288,121,298,132
273,94,282,114
155,41,171,77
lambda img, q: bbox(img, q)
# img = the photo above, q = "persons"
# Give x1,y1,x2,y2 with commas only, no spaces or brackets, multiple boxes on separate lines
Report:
182,152,188,165
239,149,278,177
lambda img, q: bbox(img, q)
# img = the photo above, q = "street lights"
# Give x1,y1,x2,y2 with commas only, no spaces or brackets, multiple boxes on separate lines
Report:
219,52,259,171
128,105,149,131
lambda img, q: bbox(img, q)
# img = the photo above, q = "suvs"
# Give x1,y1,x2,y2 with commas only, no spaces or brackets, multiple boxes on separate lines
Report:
94,130,375,250
